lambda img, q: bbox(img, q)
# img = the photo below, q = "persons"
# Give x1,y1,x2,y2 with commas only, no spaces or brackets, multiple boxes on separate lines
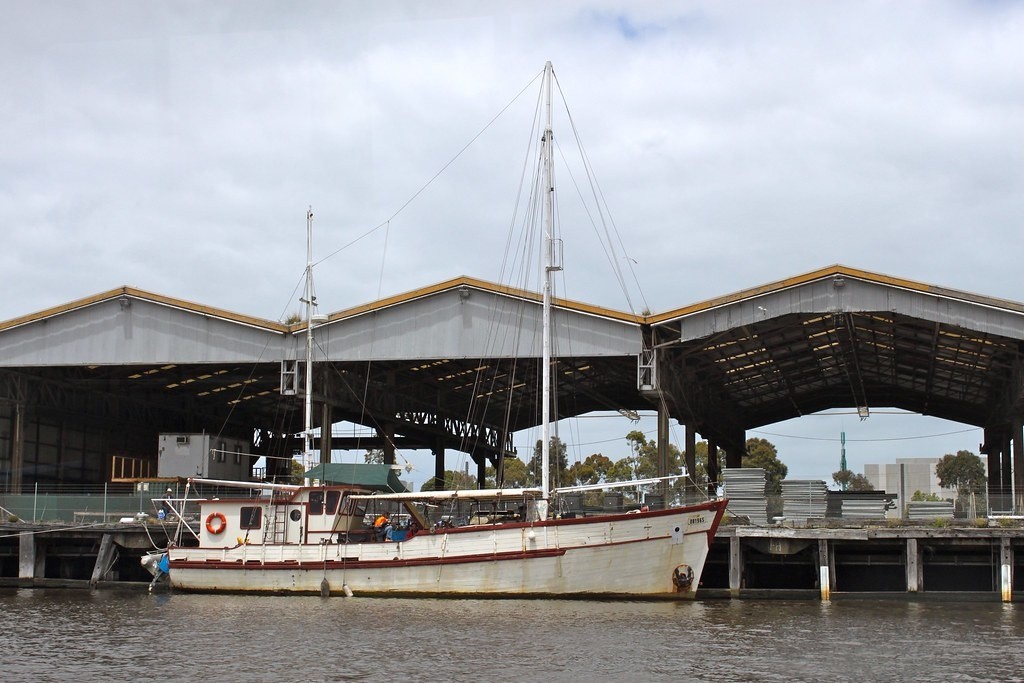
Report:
160,488,176,520
407,523,419,540
375,511,394,543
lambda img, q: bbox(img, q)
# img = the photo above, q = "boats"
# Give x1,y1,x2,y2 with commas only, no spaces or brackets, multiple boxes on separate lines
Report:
142,60,731,597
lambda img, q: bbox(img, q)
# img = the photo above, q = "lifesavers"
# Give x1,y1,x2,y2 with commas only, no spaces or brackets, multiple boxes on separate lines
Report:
206,512,226,534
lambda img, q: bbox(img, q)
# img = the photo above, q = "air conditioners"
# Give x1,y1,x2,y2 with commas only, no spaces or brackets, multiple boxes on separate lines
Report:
176,436,188,444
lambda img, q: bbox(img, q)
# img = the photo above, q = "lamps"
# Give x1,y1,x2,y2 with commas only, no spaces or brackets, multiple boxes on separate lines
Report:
118,297,132,309
459,288,469,299
834,275,846,288
